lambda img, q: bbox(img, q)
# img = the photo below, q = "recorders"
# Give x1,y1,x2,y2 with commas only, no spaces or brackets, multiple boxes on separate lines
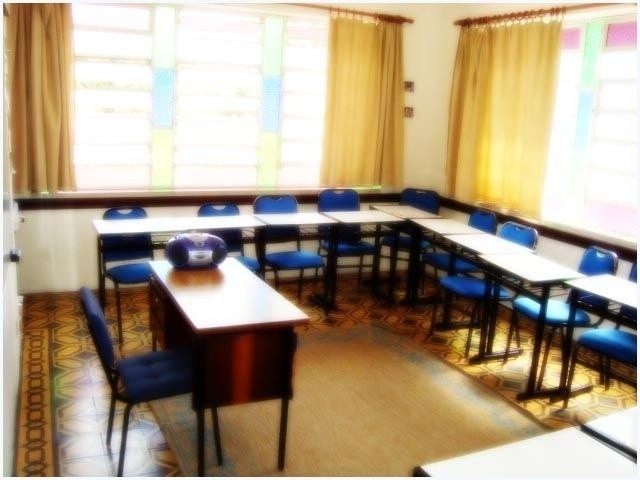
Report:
163,232,228,270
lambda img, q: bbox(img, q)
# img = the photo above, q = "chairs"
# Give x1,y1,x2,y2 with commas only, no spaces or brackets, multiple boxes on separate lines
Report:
74,187,637,476
